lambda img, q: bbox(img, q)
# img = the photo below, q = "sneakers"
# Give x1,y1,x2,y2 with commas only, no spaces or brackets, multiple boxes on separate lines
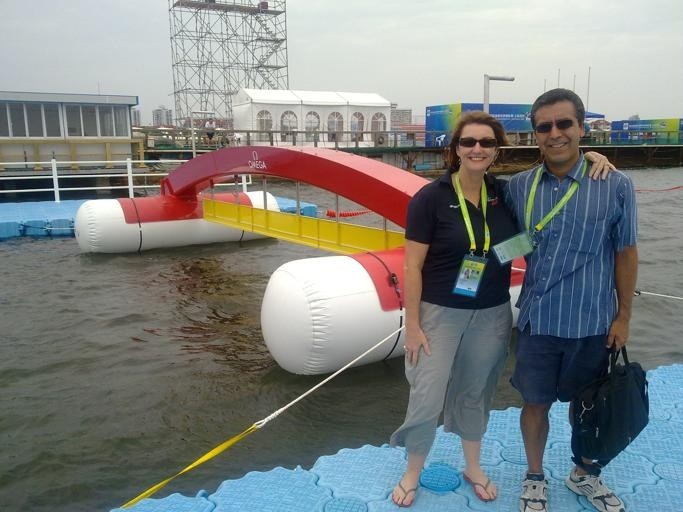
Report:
519,470,549,512
566,466,625,512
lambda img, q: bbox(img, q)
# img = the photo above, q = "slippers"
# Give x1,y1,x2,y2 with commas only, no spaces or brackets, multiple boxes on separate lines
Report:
463,470,497,502
392,481,417,507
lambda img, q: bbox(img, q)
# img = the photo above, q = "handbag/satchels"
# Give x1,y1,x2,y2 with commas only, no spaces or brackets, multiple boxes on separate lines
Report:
571,342,649,467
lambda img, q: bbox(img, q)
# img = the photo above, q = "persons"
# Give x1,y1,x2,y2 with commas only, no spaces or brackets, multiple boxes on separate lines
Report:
203,118,216,148
391,108,621,508
504,87,640,511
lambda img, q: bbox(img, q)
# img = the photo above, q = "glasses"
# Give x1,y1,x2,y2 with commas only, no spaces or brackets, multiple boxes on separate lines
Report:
535,120,572,133
460,137,497,147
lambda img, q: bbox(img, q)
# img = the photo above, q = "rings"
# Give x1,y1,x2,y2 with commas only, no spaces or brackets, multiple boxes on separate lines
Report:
604,163,610,168
402,344,408,352
